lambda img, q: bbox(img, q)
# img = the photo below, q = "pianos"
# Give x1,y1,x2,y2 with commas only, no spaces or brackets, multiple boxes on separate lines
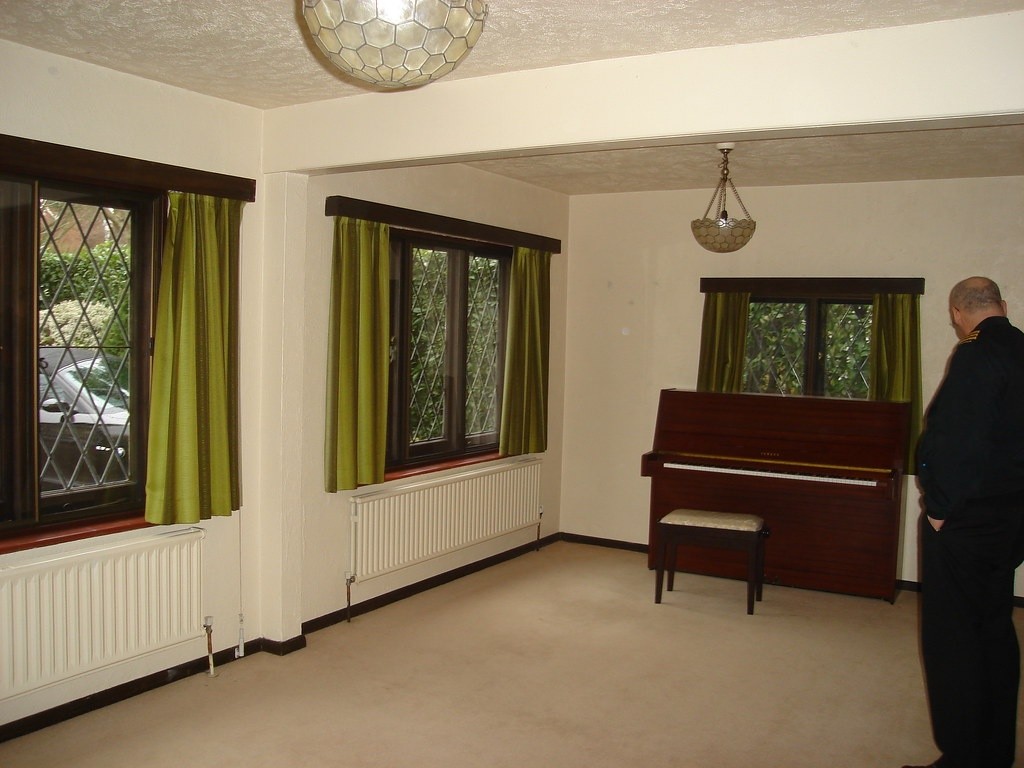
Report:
638,386,913,606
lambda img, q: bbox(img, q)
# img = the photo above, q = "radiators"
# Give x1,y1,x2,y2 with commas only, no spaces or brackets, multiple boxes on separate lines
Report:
0,526,216,706
345,458,544,623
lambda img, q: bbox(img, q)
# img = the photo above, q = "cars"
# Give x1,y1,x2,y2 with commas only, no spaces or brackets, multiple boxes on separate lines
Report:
38,347,129,491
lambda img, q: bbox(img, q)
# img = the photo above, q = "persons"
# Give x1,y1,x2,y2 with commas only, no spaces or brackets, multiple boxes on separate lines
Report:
903,277,1024,768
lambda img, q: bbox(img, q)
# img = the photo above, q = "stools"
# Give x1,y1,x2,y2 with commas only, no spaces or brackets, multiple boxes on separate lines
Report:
652,508,766,615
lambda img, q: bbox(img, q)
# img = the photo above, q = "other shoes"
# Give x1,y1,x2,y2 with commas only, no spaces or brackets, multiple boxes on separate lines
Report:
901,753,945,768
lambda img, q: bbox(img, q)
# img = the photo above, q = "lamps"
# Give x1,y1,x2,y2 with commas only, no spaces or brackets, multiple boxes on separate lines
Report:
301,0,489,90
691,143,757,254
